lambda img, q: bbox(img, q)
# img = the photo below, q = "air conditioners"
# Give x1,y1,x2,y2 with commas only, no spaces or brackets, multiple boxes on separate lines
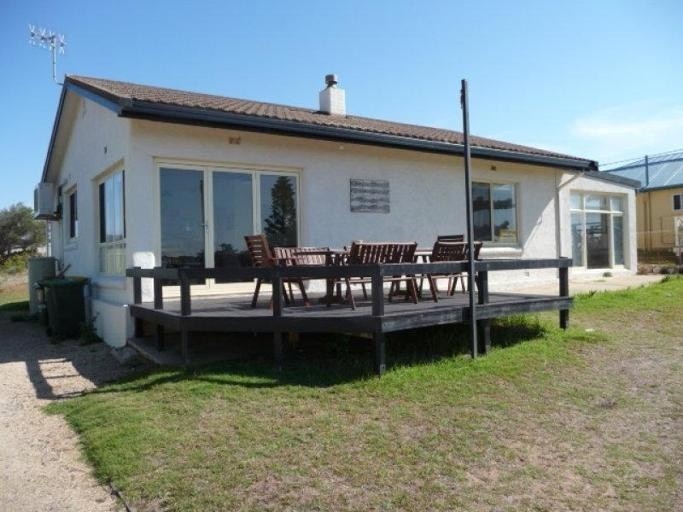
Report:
34,182,56,218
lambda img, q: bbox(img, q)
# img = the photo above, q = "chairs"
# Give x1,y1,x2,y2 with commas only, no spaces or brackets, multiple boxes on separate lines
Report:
244,234,483,311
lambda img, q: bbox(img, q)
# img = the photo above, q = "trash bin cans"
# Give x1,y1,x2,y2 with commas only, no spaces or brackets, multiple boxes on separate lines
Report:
37,276,88,342
